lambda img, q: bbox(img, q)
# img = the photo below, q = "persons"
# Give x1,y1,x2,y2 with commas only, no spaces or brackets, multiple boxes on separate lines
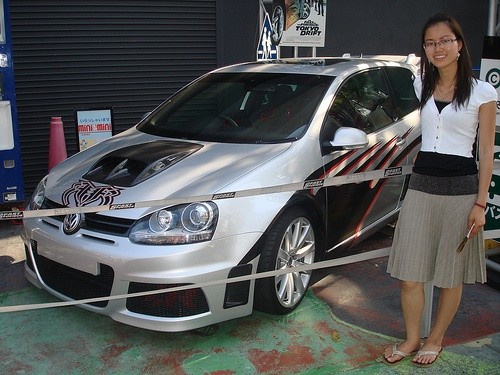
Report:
382,14,497,367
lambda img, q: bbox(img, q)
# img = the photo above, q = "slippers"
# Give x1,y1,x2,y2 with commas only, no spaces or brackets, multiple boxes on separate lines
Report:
381,342,421,366
411,348,444,368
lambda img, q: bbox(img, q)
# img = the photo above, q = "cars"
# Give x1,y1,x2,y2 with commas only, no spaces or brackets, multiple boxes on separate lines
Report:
20,52,425,334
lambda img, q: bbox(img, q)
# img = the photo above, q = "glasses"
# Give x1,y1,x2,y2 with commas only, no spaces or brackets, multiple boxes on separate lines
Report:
423,38,457,52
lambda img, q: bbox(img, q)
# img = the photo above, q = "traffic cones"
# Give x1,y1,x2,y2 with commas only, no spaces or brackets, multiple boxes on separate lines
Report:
48,116,68,170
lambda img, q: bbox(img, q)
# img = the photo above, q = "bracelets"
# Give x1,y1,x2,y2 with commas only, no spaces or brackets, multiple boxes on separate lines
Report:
475,202,485,210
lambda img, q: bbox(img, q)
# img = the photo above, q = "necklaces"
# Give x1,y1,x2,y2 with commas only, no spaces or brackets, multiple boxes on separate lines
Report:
437,85,455,100
